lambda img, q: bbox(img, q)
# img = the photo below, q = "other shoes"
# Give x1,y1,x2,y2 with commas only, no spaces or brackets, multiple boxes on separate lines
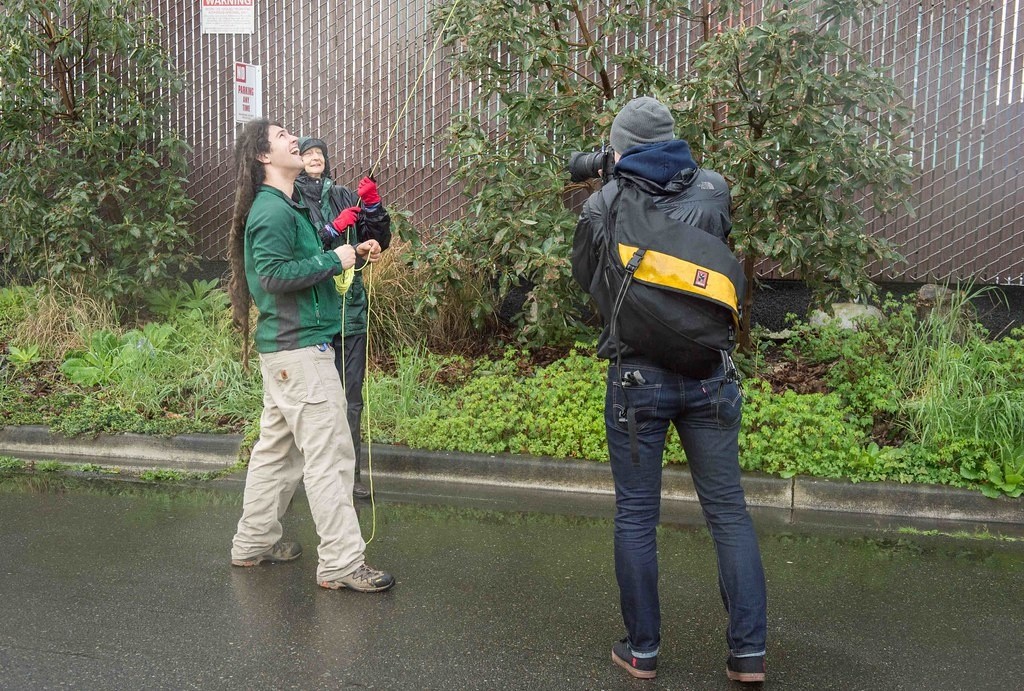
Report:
353,480,374,499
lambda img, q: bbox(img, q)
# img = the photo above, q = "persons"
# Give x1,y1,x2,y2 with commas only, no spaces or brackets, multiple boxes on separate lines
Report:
230,116,395,592
291,136,392,497
571,96,768,683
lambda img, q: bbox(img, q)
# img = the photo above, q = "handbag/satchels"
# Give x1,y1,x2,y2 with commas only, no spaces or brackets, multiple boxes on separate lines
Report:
589,179,746,378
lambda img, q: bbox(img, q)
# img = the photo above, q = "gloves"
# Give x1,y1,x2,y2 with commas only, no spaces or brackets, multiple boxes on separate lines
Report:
357,168,382,206
330,205,362,235
617,369,649,388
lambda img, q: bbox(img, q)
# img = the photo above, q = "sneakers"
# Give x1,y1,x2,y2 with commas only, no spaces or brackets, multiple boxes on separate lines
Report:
611,637,657,679
725,654,766,682
231,539,303,566
316,560,395,592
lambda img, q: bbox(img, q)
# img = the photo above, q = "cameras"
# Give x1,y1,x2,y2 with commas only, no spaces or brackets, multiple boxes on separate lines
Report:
569,145,617,184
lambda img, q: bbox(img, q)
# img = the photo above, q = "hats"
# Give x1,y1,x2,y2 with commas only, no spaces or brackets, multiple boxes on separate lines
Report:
609,96,677,155
